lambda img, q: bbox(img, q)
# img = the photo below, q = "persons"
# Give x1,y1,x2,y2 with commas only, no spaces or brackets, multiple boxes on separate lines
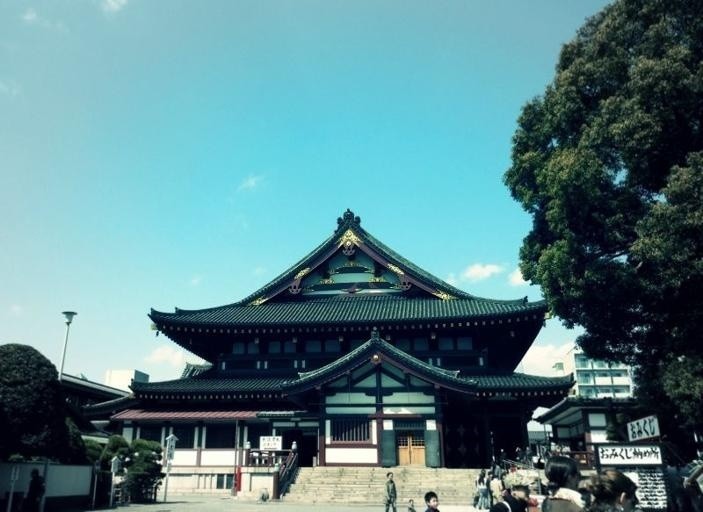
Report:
383,472,397,512
24,469,46,511
473,443,703,512
424,492,440,512
408,499,416,512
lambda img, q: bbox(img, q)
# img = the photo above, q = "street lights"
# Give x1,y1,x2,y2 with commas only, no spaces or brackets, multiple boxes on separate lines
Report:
58,309,77,382
